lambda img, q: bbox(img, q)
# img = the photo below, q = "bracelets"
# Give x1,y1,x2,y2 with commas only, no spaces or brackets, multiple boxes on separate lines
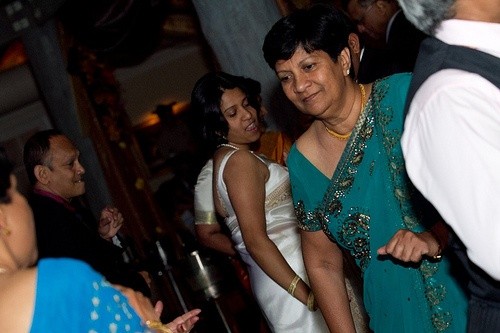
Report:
426,230,444,260
306,291,318,313
146,319,173,333
286,276,301,296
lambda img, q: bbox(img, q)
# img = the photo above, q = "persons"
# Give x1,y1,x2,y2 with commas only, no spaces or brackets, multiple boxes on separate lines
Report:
399,0,500,333
342,0,432,86
191,70,370,333
262,4,468,333
24,130,153,302
0,153,201,333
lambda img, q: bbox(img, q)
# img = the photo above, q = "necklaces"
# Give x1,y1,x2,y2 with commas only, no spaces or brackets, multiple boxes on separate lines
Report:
323,82,367,140
214,141,239,151
